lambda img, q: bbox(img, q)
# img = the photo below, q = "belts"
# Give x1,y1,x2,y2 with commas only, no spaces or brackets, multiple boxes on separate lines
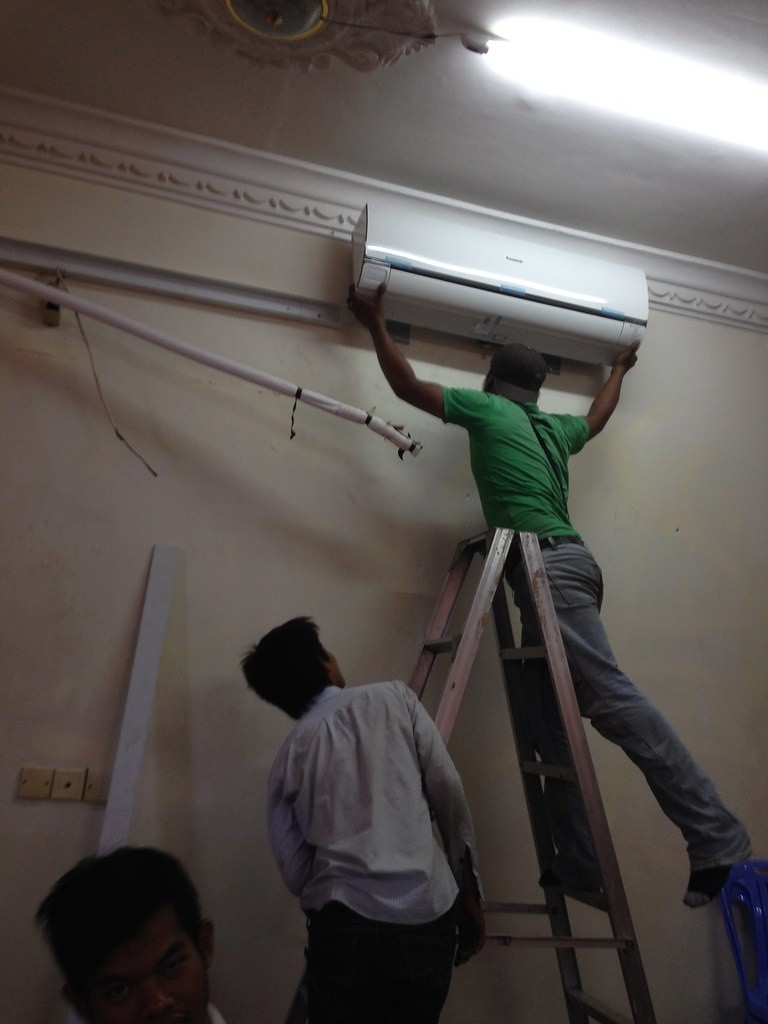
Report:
506,534,585,571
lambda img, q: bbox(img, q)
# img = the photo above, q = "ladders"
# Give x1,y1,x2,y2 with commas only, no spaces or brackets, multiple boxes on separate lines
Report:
285,527,658,1024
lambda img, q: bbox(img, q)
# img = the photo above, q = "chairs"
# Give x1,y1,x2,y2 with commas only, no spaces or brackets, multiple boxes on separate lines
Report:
719,860,768,1023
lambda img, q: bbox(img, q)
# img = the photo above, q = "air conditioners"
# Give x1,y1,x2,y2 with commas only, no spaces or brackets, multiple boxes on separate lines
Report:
349,201,650,368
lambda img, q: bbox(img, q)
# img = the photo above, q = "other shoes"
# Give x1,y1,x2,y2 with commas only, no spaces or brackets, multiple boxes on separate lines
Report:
683,864,733,907
538,856,600,892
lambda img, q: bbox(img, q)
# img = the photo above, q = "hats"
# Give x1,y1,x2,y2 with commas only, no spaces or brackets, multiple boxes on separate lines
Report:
490,343,548,393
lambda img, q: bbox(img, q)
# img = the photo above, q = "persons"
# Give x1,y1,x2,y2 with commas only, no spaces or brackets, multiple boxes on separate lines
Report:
345,282,754,911
238,615,484,1024
33,845,214,1024
